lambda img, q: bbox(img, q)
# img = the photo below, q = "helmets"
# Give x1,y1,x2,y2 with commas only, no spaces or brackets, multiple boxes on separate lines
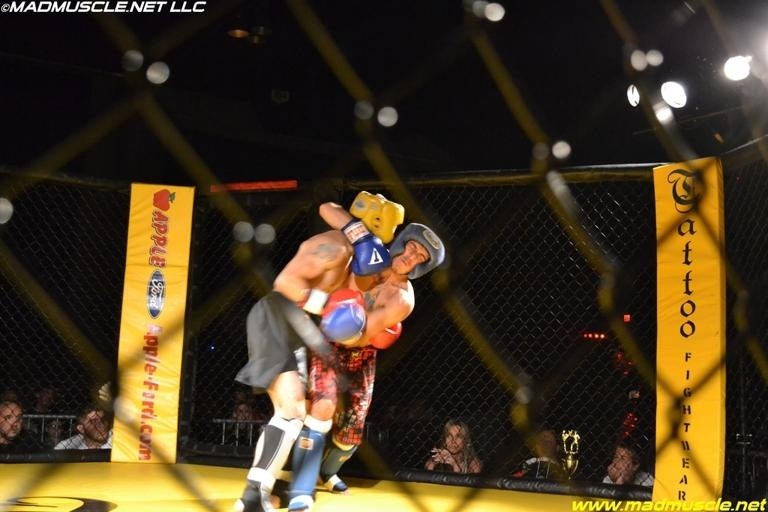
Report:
389,222,446,280
349,191,406,245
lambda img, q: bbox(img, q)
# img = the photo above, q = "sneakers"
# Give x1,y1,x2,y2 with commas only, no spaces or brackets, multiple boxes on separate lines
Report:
319,472,349,493
287,494,316,512
231,497,275,512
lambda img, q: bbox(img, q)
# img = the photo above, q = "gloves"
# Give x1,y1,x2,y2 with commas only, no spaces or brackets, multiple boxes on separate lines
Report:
369,321,403,350
319,302,366,346
342,219,391,276
301,288,367,318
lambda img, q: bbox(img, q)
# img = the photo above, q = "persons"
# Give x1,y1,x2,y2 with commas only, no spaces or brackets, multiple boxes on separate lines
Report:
512,429,573,481
424,416,482,474
0,381,266,453
223,189,406,510
283,203,449,511
602,446,651,484
